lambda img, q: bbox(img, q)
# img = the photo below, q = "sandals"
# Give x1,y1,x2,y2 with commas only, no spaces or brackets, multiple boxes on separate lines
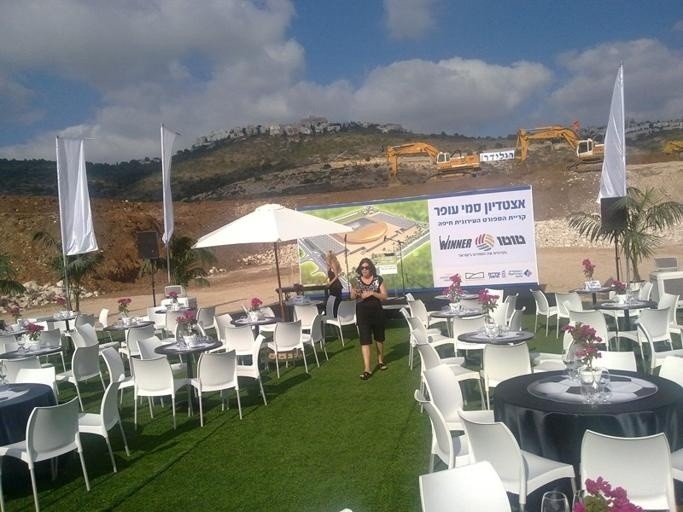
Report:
359,368,374,380
376,359,388,370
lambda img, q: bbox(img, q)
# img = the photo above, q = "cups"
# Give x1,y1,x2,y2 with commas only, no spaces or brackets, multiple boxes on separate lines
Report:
540,490,571,512
572,489,597,512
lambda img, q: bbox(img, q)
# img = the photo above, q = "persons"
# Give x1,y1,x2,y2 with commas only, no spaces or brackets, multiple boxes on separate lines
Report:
349,257,388,382
327,251,343,317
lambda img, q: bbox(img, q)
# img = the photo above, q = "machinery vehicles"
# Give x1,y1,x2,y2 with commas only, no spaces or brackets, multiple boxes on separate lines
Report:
385,141,482,190
514,125,605,174
662,138,683,161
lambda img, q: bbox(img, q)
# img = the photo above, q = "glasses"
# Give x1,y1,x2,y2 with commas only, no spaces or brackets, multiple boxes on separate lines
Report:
361,266,368,270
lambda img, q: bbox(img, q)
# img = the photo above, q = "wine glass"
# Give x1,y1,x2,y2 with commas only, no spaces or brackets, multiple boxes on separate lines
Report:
578,367,594,404
562,348,576,383
0,364,9,387
17,337,26,353
593,366,612,404
576,347,590,381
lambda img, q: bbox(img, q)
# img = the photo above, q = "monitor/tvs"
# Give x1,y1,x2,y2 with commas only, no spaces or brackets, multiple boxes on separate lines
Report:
648,271,683,306
165,285,186,296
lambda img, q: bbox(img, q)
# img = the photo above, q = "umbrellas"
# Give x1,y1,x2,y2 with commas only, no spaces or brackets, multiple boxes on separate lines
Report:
190,202,353,323
